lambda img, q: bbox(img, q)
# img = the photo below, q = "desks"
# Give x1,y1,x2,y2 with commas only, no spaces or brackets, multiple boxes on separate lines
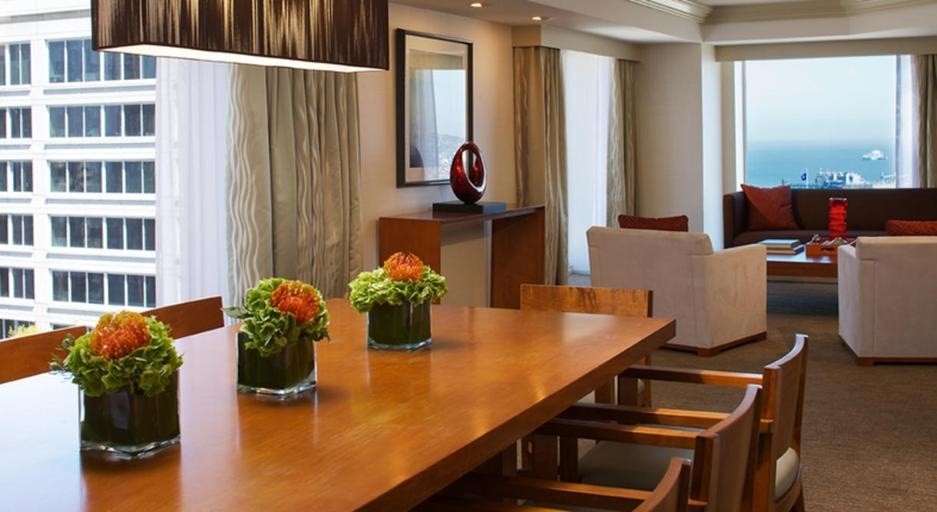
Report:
378,202,546,310
1,297,676,512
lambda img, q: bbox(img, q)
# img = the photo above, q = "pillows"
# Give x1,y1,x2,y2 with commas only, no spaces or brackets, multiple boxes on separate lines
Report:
887,218,937,236
741,184,799,229
617,214,688,231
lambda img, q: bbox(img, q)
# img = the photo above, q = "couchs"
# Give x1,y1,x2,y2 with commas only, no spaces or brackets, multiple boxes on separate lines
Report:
723,188,937,278
587,226,768,357
837,236,937,367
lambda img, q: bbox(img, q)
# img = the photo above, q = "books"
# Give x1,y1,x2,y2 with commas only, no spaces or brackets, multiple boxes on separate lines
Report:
757,238,805,256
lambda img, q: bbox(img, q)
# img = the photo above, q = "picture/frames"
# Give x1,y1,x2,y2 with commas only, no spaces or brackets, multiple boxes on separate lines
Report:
394,28,475,186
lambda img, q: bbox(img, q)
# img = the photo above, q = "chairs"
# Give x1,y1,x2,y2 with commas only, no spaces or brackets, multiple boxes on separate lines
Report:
139,295,226,342
519,285,652,408
0,325,90,386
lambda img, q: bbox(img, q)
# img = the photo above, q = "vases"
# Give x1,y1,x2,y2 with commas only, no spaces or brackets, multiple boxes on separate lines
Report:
367,303,432,352
237,334,317,398
78,371,181,460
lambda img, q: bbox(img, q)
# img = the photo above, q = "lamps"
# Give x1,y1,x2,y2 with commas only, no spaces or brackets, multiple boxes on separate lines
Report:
91,0,390,76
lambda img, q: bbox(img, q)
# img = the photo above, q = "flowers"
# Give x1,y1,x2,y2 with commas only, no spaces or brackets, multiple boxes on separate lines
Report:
347,251,447,313
47,309,181,400
220,276,331,360
